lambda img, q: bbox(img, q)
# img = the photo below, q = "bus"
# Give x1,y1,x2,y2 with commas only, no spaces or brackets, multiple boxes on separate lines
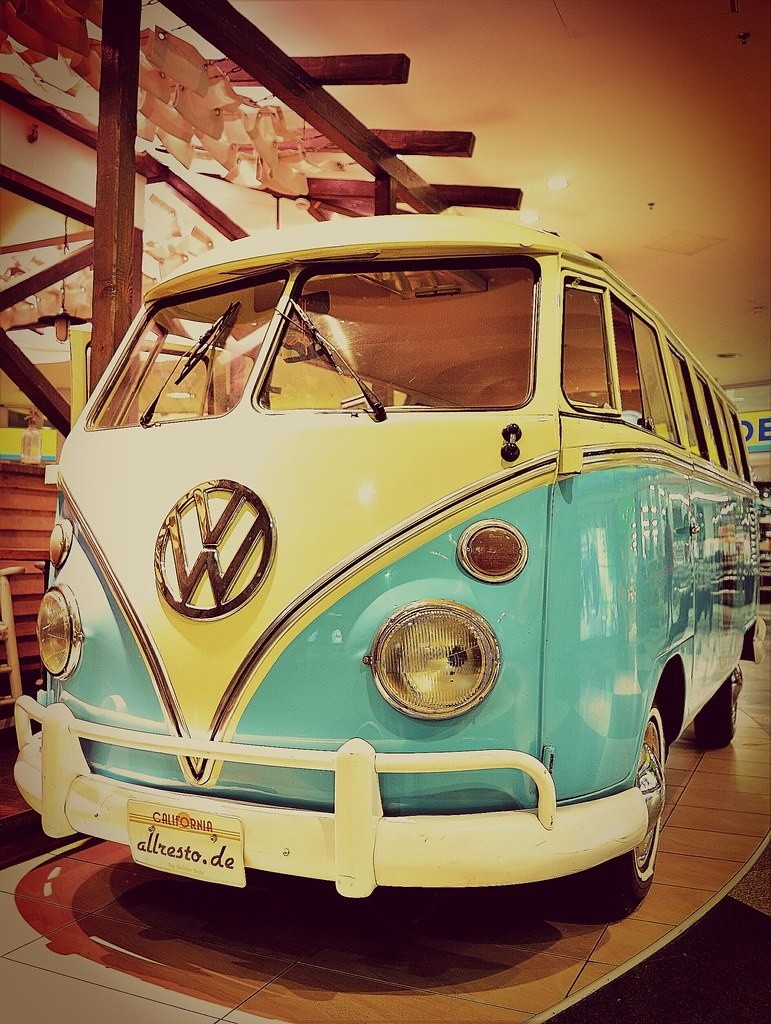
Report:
15,215,759,920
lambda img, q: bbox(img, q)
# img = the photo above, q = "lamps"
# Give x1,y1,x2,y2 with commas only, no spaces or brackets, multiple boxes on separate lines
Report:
37,215,88,341
